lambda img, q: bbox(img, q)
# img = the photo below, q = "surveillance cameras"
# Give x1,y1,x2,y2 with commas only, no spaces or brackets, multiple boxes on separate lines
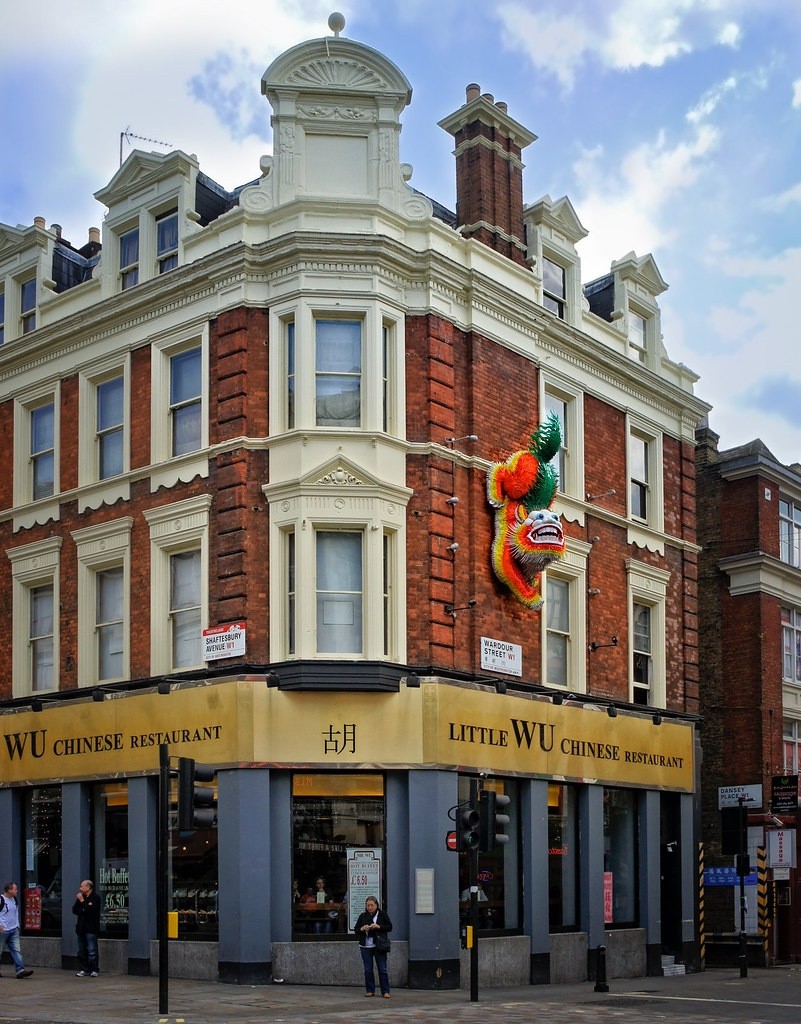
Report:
771,816,783,826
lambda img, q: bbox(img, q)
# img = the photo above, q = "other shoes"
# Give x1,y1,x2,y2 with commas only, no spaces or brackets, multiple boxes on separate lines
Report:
384,993,390,998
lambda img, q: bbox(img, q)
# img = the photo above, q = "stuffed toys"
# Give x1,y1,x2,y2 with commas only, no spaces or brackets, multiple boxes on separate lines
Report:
486,415,566,611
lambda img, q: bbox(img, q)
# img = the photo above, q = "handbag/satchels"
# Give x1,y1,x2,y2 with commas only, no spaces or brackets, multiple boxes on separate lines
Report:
376,935,391,953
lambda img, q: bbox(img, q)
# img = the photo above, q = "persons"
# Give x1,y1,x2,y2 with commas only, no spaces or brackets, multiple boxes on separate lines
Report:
292,874,334,934
354,897,392,998
0,882,33,979
72,880,101,977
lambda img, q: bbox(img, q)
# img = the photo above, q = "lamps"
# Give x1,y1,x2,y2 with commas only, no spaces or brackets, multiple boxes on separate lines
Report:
158,678,204,695
593,535,600,542
444,435,478,443
92,687,124,702
589,636,617,651
531,690,563,705
473,678,506,694
31,698,60,713
586,489,616,501
444,599,476,614
587,588,600,595
446,496,458,507
406,671,421,688
630,711,662,725
447,541,459,553
581,702,617,718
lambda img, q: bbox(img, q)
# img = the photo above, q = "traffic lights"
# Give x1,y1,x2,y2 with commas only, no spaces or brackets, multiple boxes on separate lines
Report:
480,790,510,848
455,808,482,852
178,757,215,832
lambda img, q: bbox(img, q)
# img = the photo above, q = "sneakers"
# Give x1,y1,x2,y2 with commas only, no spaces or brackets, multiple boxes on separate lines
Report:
76,971,98,977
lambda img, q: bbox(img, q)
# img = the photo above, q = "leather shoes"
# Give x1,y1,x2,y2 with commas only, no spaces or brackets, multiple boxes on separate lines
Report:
365,992,375,997
17,970,33,978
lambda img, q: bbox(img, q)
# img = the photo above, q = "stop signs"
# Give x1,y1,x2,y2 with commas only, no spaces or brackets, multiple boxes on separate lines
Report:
447,832,456,850
